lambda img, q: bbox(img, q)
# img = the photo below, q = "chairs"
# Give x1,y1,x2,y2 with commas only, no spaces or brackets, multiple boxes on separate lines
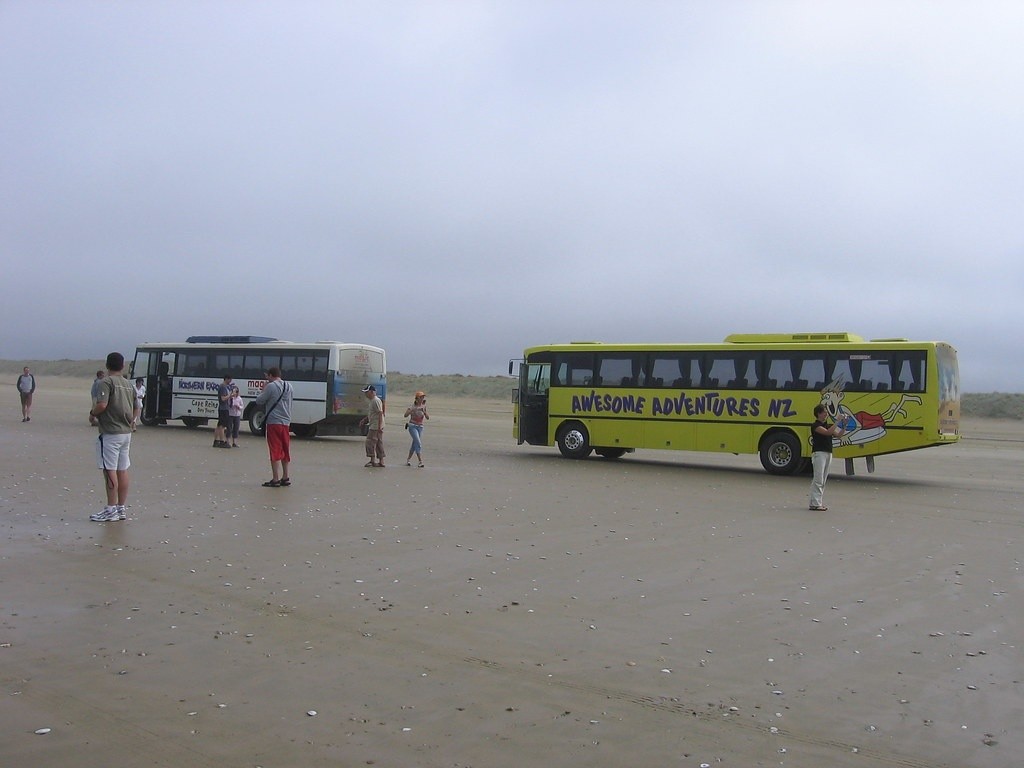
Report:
587,374,918,390
182,367,325,380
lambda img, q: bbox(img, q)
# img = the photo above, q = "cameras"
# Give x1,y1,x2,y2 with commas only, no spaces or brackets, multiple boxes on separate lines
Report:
844,414,848,419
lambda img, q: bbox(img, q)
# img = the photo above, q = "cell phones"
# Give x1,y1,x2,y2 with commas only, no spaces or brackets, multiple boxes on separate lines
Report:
422,400,426,405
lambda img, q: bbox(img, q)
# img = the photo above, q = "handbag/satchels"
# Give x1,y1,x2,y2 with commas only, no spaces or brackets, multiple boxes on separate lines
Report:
258,421,266,437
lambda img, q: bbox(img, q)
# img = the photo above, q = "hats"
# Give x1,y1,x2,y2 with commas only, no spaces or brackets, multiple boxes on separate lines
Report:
362,385,376,393
415,392,425,398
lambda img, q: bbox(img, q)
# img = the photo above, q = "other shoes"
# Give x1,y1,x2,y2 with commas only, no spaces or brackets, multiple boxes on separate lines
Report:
278,478,292,486
132,422,136,431
809,506,827,511
218,441,226,448
213,440,220,447
375,463,385,467
407,459,411,465
418,461,424,467
91,421,99,426
224,441,232,448
232,443,240,448
264,479,281,487
23,417,30,422
365,462,375,467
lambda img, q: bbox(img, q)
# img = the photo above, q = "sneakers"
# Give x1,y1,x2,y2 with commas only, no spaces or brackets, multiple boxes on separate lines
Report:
90,506,119,521
117,505,126,519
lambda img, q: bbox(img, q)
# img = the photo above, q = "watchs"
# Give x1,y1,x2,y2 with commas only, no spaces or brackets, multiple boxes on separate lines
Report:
90,409,95,416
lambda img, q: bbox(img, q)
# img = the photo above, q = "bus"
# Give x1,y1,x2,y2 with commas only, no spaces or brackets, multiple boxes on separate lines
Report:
128,335,387,438
508,332,961,475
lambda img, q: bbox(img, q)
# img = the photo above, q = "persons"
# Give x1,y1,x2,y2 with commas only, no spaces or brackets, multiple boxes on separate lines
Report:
808,404,848,510
89,352,141,521
16,367,35,422
404,392,430,467
360,385,387,467
255,367,293,486
90,370,146,432
213,373,244,447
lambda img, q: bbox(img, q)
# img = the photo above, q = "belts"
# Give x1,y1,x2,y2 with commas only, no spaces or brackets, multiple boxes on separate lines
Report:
410,423,423,428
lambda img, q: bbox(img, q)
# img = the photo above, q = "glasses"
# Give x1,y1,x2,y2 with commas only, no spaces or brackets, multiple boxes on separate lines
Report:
236,391,239,393
